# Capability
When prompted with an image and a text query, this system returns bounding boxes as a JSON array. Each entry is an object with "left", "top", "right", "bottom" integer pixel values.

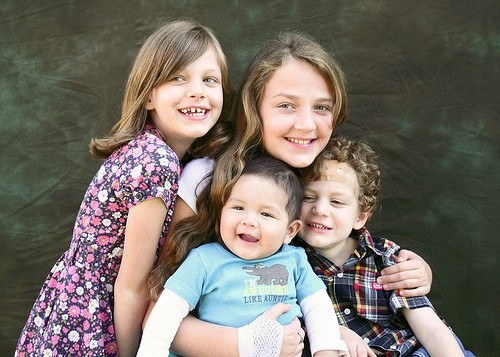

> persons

[
  {"left": 142, "top": 31, "right": 432, "bottom": 357},
  {"left": 136, "top": 159, "right": 349, "bottom": 357},
  {"left": 289, "top": 136, "right": 474, "bottom": 357},
  {"left": 13, "top": 19, "right": 229, "bottom": 357}
]
